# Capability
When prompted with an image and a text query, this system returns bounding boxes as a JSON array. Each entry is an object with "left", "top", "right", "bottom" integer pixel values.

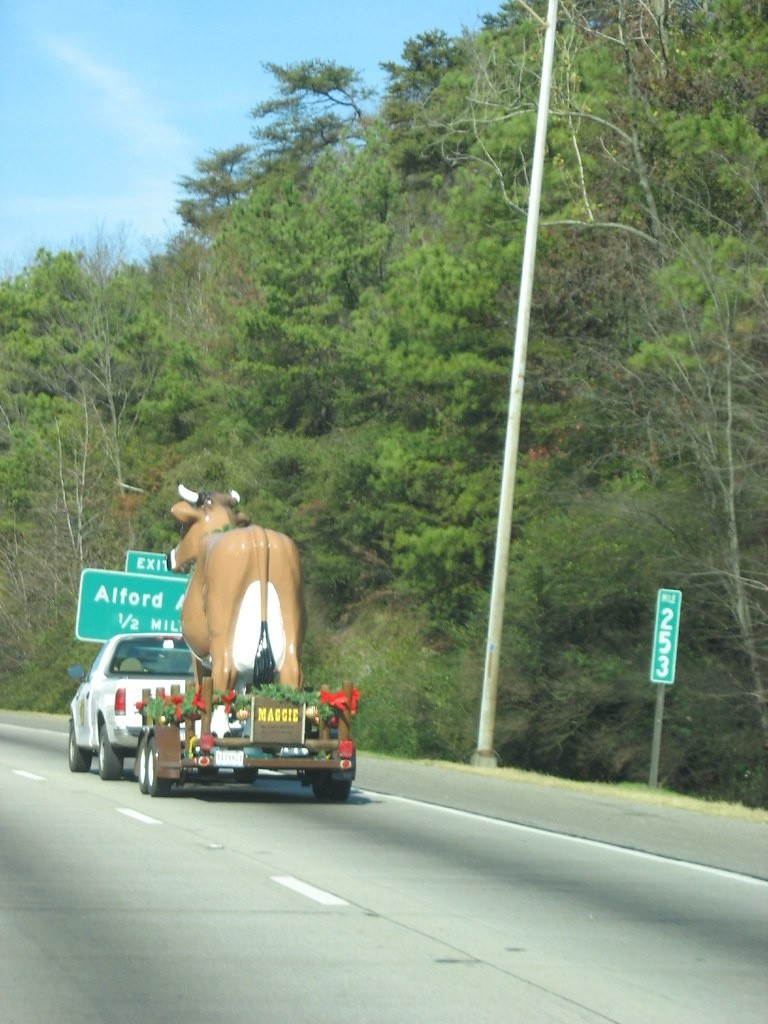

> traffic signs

[{"left": 75, "top": 550, "right": 191, "bottom": 644}]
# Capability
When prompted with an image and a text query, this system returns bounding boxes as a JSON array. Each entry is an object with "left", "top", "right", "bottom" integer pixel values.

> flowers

[{"left": 134, "top": 683, "right": 361, "bottom": 750}]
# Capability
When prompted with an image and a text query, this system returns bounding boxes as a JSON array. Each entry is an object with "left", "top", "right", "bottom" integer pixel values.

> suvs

[{"left": 66, "top": 632, "right": 261, "bottom": 784}]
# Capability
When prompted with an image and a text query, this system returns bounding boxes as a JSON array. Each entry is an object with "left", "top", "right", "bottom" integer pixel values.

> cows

[{"left": 165, "top": 482, "right": 303, "bottom": 692}]
